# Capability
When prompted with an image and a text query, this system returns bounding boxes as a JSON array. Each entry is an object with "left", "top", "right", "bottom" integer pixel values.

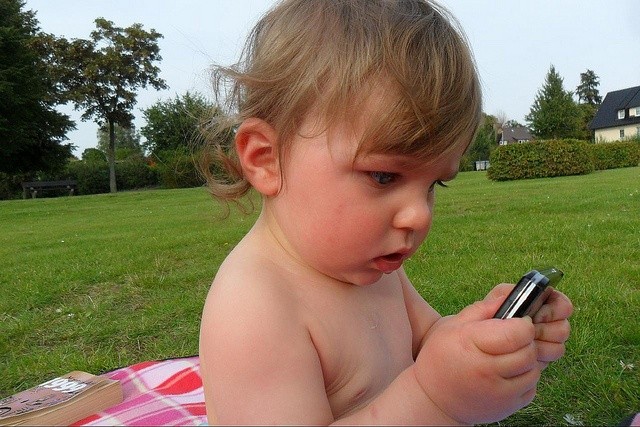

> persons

[{"left": 181, "top": 1, "right": 574, "bottom": 426}]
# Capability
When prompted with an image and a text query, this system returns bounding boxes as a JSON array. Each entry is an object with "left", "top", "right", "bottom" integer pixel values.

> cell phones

[{"left": 492, "top": 267, "right": 564, "bottom": 320}]
